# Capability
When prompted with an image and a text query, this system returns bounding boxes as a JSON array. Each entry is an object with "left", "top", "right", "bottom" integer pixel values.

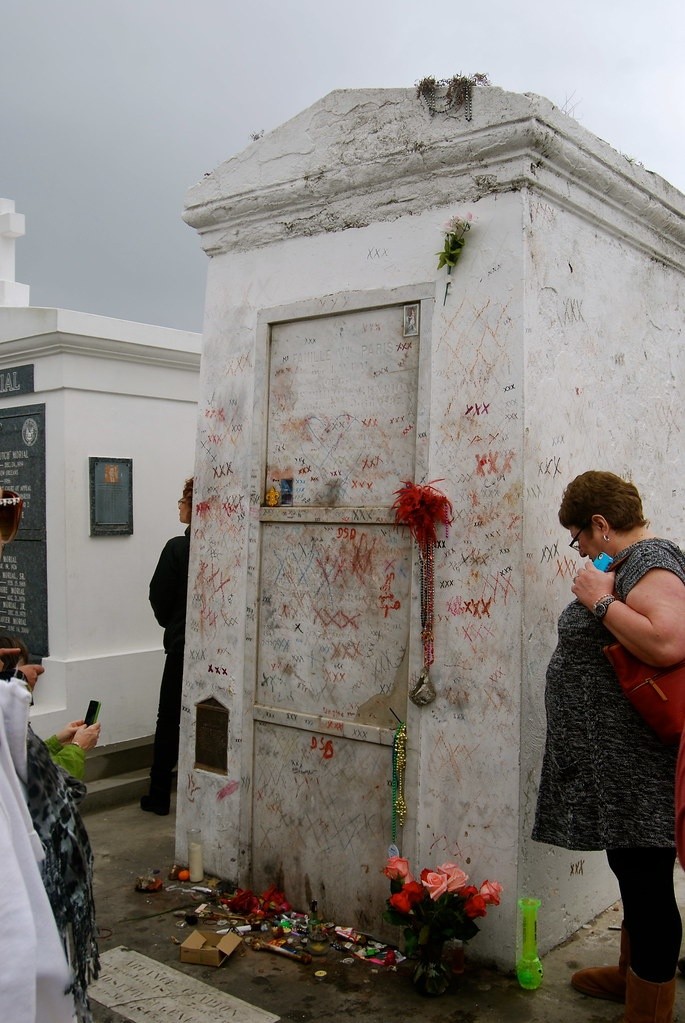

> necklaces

[
  {"left": 388, "top": 722, "right": 408, "bottom": 857},
  {"left": 593, "top": 594, "right": 614, "bottom": 617},
  {"left": 409, "top": 540, "right": 437, "bottom": 706}
]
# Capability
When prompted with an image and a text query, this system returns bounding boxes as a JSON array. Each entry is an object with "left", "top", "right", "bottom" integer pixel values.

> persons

[
  {"left": 0, "top": 488, "right": 102, "bottom": 1023},
  {"left": 140, "top": 478, "right": 195, "bottom": 814},
  {"left": 531, "top": 469, "right": 685, "bottom": 1023}
]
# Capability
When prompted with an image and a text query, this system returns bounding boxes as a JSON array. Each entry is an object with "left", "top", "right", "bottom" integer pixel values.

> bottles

[{"left": 518, "top": 897, "right": 544, "bottom": 990}]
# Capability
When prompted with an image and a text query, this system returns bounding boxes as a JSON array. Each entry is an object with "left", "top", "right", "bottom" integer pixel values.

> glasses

[{"left": 569, "top": 520, "right": 592, "bottom": 551}]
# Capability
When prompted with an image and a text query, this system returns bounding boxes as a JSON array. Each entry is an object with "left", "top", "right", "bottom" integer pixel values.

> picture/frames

[{"left": 89, "top": 456, "right": 134, "bottom": 535}]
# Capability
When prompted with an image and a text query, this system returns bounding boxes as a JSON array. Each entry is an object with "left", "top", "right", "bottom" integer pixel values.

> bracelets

[{"left": 70, "top": 742, "right": 81, "bottom": 748}]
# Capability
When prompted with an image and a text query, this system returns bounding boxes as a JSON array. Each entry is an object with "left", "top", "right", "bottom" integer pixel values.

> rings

[{"left": 572, "top": 575, "right": 579, "bottom": 584}]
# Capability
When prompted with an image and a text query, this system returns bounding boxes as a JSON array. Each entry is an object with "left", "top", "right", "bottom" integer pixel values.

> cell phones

[
  {"left": 28, "top": 655, "right": 42, "bottom": 666},
  {"left": 83, "top": 700, "right": 101, "bottom": 728},
  {"left": 593, "top": 552, "right": 613, "bottom": 572}
]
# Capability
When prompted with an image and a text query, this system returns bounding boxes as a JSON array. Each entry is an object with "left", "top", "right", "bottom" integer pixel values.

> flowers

[
  {"left": 438, "top": 208, "right": 472, "bottom": 305},
  {"left": 383, "top": 855, "right": 502, "bottom": 959}
]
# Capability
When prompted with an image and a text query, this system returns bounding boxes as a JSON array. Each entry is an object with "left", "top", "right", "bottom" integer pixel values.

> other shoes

[
  {"left": 140, "top": 796, "right": 170, "bottom": 815},
  {"left": 625, "top": 966, "right": 675, "bottom": 1023},
  {"left": 572, "top": 919, "right": 629, "bottom": 1001}
]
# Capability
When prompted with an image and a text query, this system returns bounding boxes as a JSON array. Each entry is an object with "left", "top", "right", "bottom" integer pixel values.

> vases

[
  {"left": 514, "top": 897, "right": 543, "bottom": 990},
  {"left": 408, "top": 935, "right": 454, "bottom": 997}
]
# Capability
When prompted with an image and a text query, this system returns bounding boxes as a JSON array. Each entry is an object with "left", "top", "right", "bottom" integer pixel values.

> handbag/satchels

[{"left": 603, "top": 643, "right": 685, "bottom": 736}]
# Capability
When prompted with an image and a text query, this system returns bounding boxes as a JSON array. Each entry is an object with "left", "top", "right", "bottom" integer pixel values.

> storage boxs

[{"left": 180, "top": 929, "right": 243, "bottom": 968}]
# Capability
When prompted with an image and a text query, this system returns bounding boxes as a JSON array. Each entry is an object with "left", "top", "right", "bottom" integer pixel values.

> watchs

[{"left": 594, "top": 597, "right": 619, "bottom": 622}]
